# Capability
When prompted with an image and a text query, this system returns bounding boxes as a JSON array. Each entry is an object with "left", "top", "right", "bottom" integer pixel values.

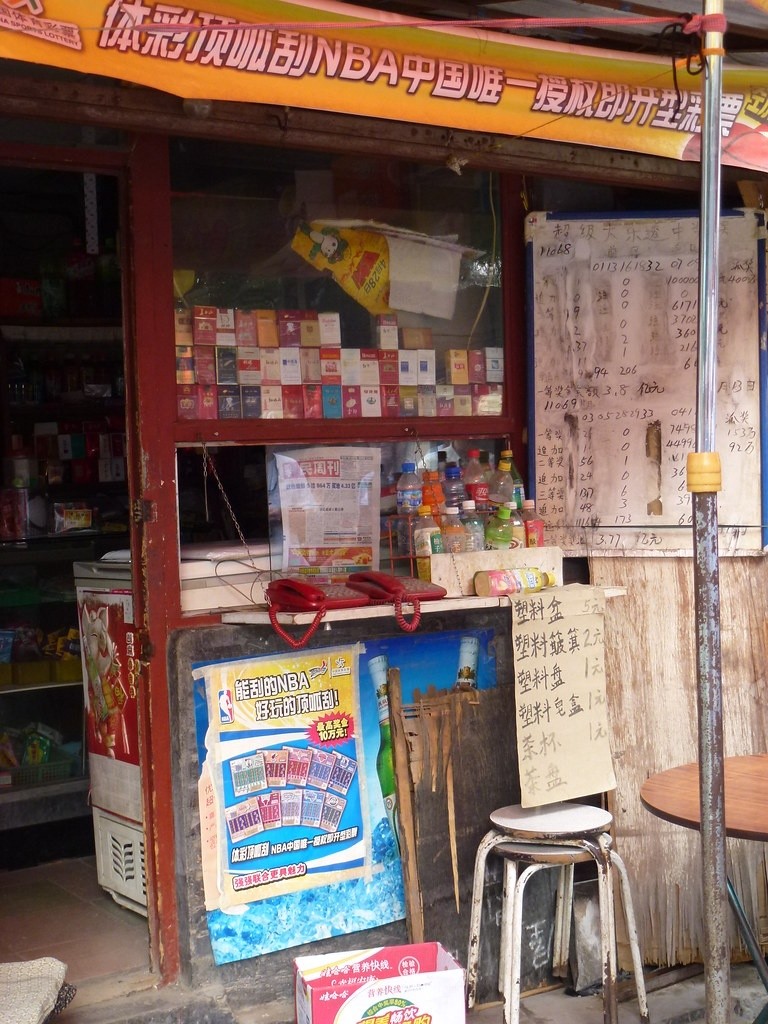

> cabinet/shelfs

[{"left": 0, "top": 317, "right": 130, "bottom": 835}]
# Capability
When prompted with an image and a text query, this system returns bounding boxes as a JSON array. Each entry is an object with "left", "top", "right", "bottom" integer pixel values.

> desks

[{"left": 640, "top": 752, "right": 768, "bottom": 1024}]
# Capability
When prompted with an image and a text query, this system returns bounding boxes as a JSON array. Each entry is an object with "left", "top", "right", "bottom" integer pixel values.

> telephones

[
  {"left": 346, "top": 571, "right": 447, "bottom": 603},
  {"left": 266, "top": 579, "right": 369, "bottom": 612}
]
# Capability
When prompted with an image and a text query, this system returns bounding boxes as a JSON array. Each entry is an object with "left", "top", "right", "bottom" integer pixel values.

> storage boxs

[
  {"left": 415, "top": 545, "right": 564, "bottom": 598},
  {"left": 173, "top": 304, "right": 507, "bottom": 419},
  {"left": 291, "top": 941, "right": 468, "bottom": 1024},
  {"left": 0, "top": 657, "right": 81, "bottom": 691},
  {"left": 0, "top": 746, "right": 75, "bottom": 795}
]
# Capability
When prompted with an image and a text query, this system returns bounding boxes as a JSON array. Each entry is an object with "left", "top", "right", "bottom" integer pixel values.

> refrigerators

[{"left": 73, "top": 537, "right": 414, "bottom": 917}]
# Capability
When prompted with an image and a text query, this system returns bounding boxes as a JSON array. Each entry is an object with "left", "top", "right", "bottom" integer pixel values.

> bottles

[
  {"left": 521, "top": 499, "right": 544, "bottom": 548},
  {"left": 422, "top": 471, "right": 443, "bottom": 522},
  {"left": 502, "top": 450, "right": 526, "bottom": 509},
  {"left": 367, "top": 656, "right": 419, "bottom": 853},
  {"left": 484, "top": 506, "right": 511, "bottom": 549},
  {"left": 444, "top": 467, "right": 466, "bottom": 511},
  {"left": 395, "top": 464, "right": 420, "bottom": 552},
  {"left": 464, "top": 448, "right": 489, "bottom": 510},
  {"left": 460, "top": 499, "right": 484, "bottom": 551},
  {"left": 490, "top": 459, "right": 514, "bottom": 508},
  {"left": 441, "top": 508, "right": 461, "bottom": 553},
  {"left": 476, "top": 567, "right": 555, "bottom": 595},
  {"left": 504, "top": 502, "right": 526, "bottom": 548},
  {"left": 413, "top": 506, "right": 441, "bottom": 582}
]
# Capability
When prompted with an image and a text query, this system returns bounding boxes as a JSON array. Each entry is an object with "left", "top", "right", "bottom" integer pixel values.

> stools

[{"left": 466, "top": 803, "right": 653, "bottom": 1024}]
